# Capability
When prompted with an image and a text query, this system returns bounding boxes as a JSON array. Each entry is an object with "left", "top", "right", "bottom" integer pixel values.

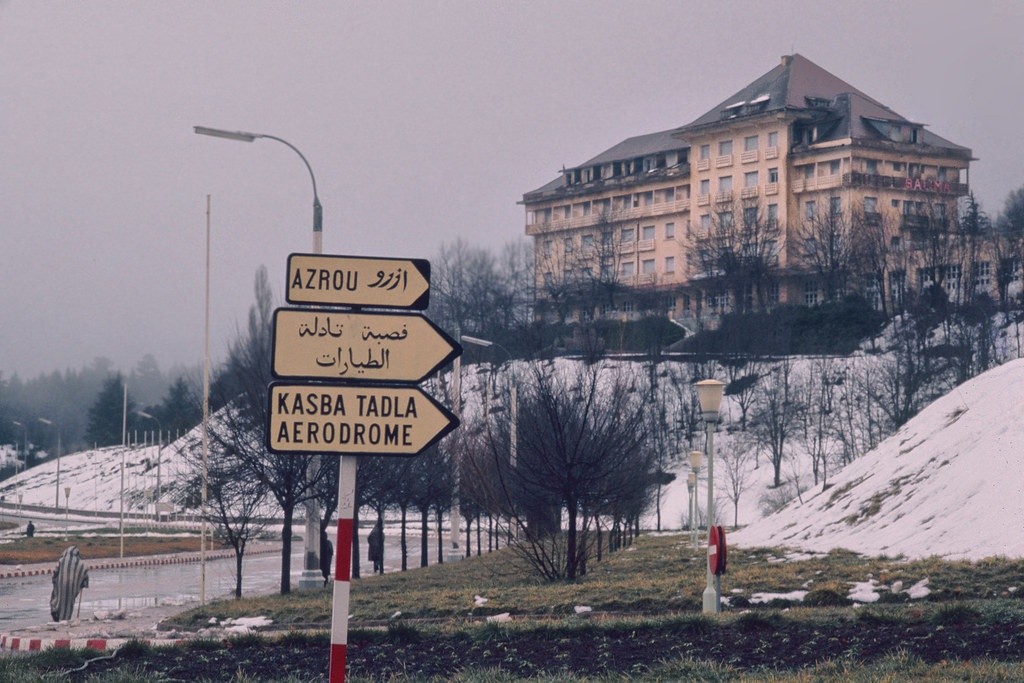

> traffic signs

[{"left": 266, "top": 246, "right": 463, "bottom": 456}]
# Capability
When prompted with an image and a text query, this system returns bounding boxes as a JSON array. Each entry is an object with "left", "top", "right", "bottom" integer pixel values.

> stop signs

[{"left": 706, "top": 524, "right": 721, "bottom": 576}]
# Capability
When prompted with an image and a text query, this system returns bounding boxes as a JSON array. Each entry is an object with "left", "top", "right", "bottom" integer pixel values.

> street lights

[
  {"left": 693, "top": 377, "right": 729, "bottom": 619},
  {"left": 459, "top": 333, "right": 523, "bottom": 552},
  {"left": 6, "top": 406, "right": 169, "bottom": 547},
  {"left": 683, "top": 448, "right": 703, "bottom": 556},
  {"left": 193, "top": 122, "right": 328, "bottom": 592}
]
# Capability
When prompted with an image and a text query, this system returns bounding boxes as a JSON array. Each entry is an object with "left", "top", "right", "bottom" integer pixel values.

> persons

[
  {"left": 320, "top": 531, "right": 334, "bottom": 586},
  {"left": 50, "top": 545, "right": 89, "bottom": 622},
  {"left": 26, "top": 520, "right": 34, "bottom": 537},
  {"left": 368, "top": 523, "right": 385, "bottom": 573}
]
